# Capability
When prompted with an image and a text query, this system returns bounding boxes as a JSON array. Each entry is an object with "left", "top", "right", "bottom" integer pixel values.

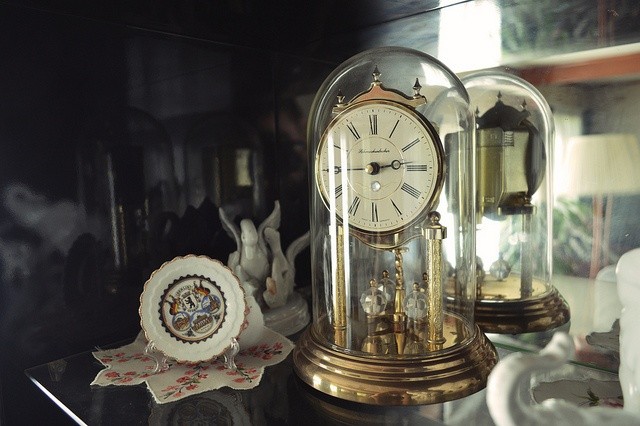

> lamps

[{"left": 557, "top": 134, "right": 639, "bottom": 288}]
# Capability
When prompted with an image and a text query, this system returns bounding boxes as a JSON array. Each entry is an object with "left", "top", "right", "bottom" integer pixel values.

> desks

[{"left": 26, "top": 290, "right": 616, "bottom": 426}]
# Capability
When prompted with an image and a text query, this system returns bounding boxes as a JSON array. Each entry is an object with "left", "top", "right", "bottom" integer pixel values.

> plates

[{"left": 137, "top": 252, "right": 250, "bottom": 367}]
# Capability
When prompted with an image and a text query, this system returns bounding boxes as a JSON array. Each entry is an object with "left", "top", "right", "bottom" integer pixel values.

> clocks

[
  {"left": 422, "top": 70, "right": 570, "bottom": 335},
  {"left": 291, "top": 46, "right": 499, "bottom": 405}
]
